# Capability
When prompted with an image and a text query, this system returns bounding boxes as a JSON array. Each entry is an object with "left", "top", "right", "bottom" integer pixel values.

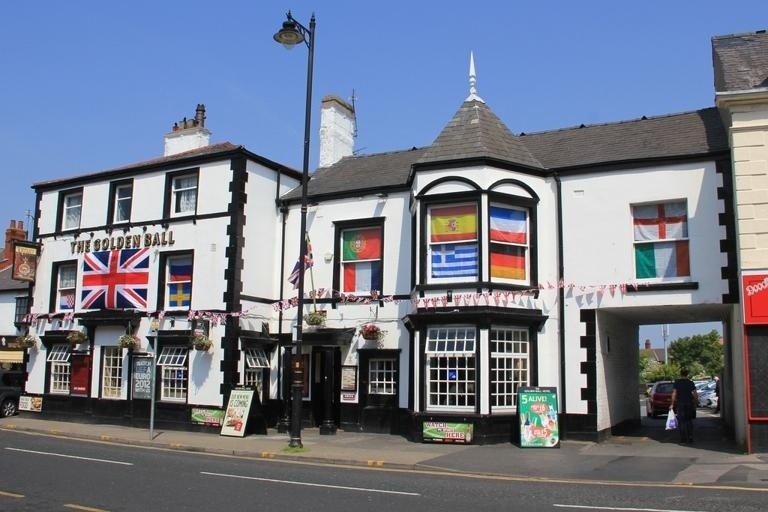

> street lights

[{"left": 273, "top": 9, "right": 316, "bottom": 450}]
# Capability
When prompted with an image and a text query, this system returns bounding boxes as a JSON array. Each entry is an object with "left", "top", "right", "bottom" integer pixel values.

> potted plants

[
  {"left": 192, "top": 334, "right": 213, "bottom": 351},
  {"left": 304, "top": 313, "right": 323, "bottom": 325}
]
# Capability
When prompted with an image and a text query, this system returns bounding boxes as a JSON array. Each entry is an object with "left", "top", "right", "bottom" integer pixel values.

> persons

[
  {"left": 669, "top": 370, "right": 698, "bottom": 445},
  {"left": 712, "top": 374, "right": 723, "bottom": 415}
]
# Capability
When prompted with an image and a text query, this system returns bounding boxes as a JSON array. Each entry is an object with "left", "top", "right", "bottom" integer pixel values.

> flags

[
  {"left": 428, "top": 205, "right": 526, "bottom": 280},
  {"left": 272, "top": 296, "right": 298, "bottom": 313},
  {"left": 342, "top": 226, "right": 383, "bottom": 260},
  {"left": 287, "top": 234, "right": 314, "bottom": 288},
  {"left": 58, "top": 248, "right": 192, "bottom": 312},
  {"left": 343, "top": 263, "right": 383, "bottom": 295},
  {"left": 634, "top": 202, "right": 687, "bottom": 238},
  {"left": 635, "top": 242, "right": 691, "bottom": 278}
]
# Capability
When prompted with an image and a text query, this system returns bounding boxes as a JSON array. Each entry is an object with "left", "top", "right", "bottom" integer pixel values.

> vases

[
  {"left": 18, "top": 341, "right": 33, "bottom": 348},
  {"left": 363, "top": 331, "right": 378, "bottom": 340},
  {"left": 122, "top": 343, "right": 136, "bottom": 348}
]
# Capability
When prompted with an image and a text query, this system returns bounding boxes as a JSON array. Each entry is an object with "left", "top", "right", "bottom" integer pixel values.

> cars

[
  {"left": 646, "top": 381, "right": 698, "bottom": 418},
  {"left": 694, "top": 375, "right": 719, "bottom": 409},
  {"left": 0, "top": 369, "right": 24, "bottom": 416}
]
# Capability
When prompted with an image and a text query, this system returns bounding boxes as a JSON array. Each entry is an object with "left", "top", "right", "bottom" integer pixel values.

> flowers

[
  {"left": 119, "top": 335, "right": 139, "bottom": 346},
  {"left": 359, "top": 323, "right": 381, "bottom": 336},
  {"left": 16, "top": 333, "right": 37, "bottom": 345}
]
